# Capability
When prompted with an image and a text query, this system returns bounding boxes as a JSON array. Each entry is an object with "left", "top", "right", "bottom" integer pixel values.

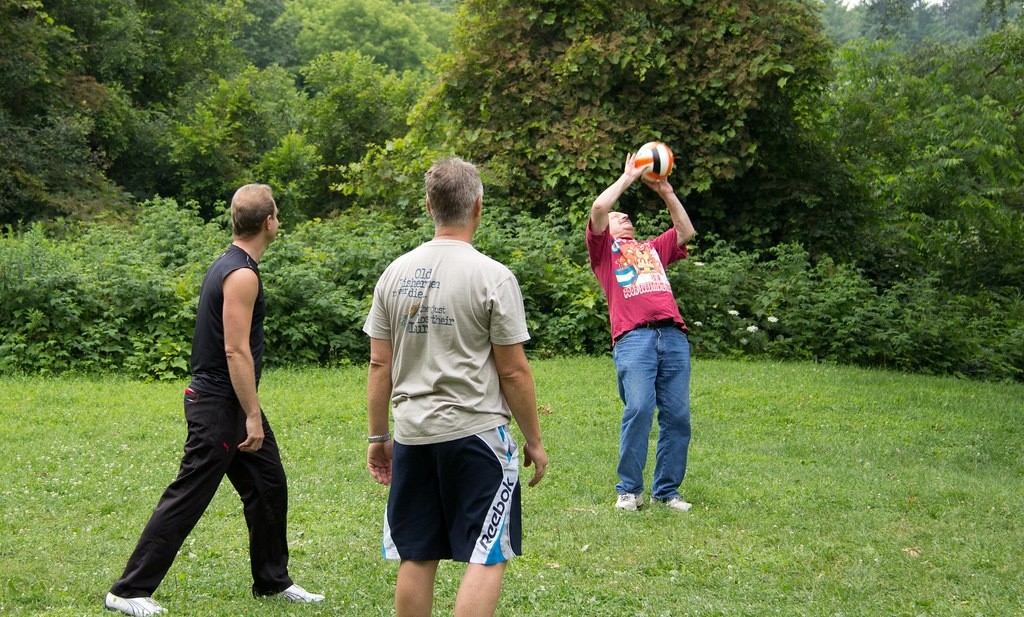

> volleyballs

[{"left": 634, "top": 141, "right": 675, "bottom": 183}]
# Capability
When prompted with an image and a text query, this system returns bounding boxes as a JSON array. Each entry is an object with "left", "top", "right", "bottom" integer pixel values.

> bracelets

[{"left": 369, "top": 434, "right": 391, "bottom": 443}]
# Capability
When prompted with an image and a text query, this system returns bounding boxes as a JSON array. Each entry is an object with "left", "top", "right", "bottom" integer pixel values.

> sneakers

[
  {"left": 253, "top": 583, "right": 326, "bottom": 603},
  {"left": 615, "top": 493, "right": 643, "bottom": 512},
  {"left": 105, "top": 592, "right": 168, "bottom": 617},
  {"left": 649, "top": 496, "right": 692, "bottom": 512}
]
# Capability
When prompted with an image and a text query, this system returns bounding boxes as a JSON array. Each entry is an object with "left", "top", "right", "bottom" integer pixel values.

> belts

[{"left": 637, "top": 321, "right": 674, "bottom": 329}]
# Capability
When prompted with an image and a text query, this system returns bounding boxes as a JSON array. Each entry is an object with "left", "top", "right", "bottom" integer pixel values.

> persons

[
  {"left": 103, "top": 184, "right": 325, "bottom": 617},
  {"left": 364, "top": 157, "right": 547, "bottom": 617},
  {"left": 586, "top": 152, "right": 696, "bottom": 512}
]
